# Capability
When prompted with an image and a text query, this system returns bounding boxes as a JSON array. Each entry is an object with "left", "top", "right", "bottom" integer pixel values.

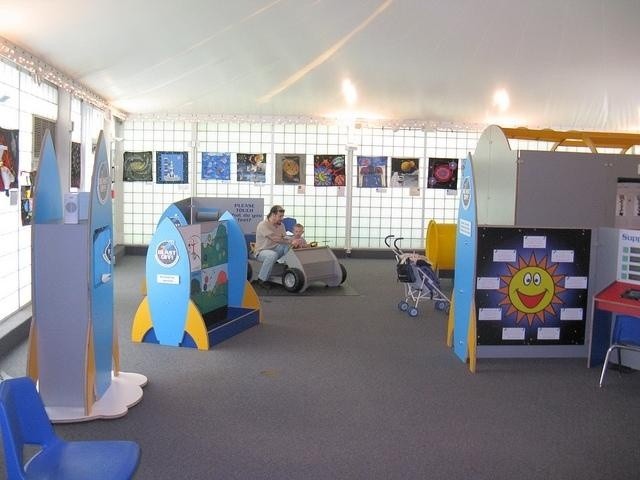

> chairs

[
  {"left": 0, "top": 375, "right": 140, "bottom": 480},
  {"left": 598, "top": 314, "right": 640, "bottom": 387}
]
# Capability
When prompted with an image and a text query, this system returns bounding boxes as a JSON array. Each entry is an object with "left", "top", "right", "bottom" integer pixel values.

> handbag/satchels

[{"left": 397, "top": 263, "right": 416, "bottom": 283}]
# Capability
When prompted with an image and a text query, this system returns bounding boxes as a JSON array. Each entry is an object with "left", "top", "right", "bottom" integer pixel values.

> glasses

[{"left": 275, "top": 209, "right": 285, "bottom": 212}]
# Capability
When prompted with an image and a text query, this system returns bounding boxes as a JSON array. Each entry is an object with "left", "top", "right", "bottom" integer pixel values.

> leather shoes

[{"left": 257, "top": 279, "right": 272, "bottom": 290}]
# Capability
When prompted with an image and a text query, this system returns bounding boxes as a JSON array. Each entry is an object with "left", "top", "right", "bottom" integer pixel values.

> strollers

[{"left": 385, "top": 235, "right": 455, "bottom": 321}]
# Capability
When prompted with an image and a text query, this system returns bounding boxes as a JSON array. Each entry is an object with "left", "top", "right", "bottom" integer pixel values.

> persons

[{"left": 254, "top": 205, "right": 308, "bottom": 290}]
